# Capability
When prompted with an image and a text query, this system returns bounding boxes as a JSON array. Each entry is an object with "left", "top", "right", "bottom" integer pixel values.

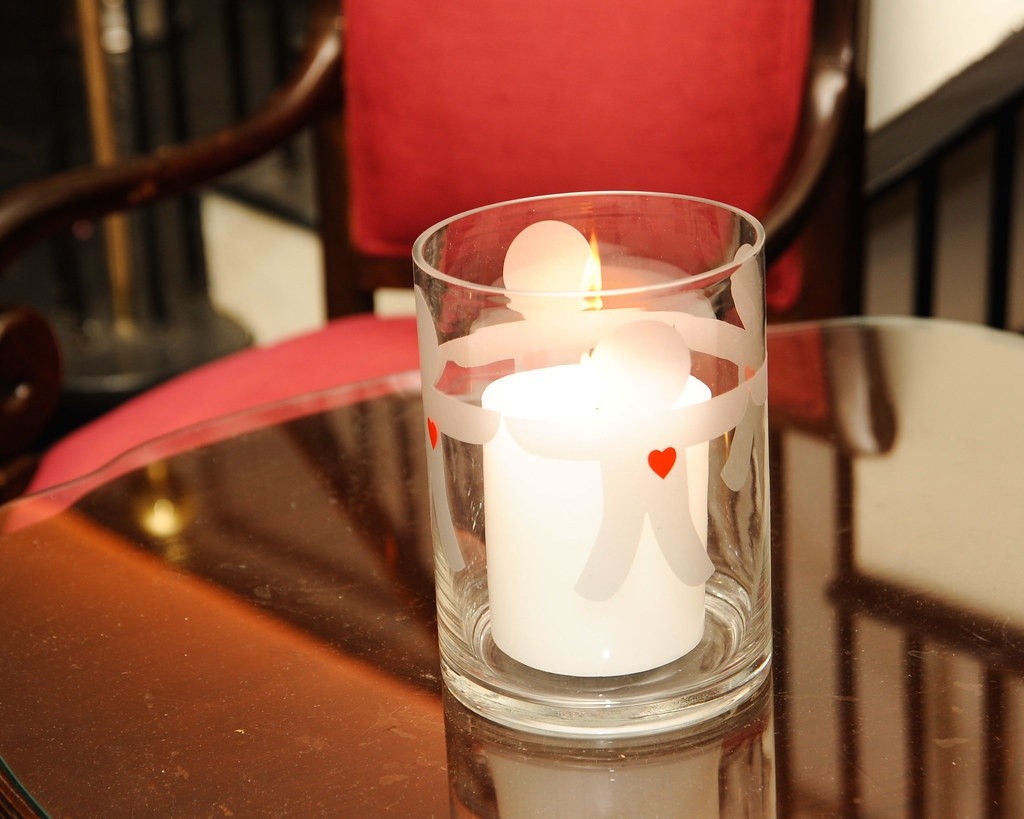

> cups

[{"left": 413, "top": 190, "right": 771, "bottom": 738}]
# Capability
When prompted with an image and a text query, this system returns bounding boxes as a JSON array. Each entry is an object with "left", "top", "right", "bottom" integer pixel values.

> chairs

[{"left": 0, "top": 0, "right": 870, "bottom": 536}]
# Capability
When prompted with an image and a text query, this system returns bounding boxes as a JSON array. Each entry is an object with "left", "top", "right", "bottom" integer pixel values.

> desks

[{"left": 0, "top": 312, "right": 1024, "bottom": 817}]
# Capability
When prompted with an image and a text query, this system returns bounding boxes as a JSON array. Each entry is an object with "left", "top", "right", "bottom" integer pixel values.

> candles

[{"left": 484, "top": 229, "right": 718, "bottom": 680}]
